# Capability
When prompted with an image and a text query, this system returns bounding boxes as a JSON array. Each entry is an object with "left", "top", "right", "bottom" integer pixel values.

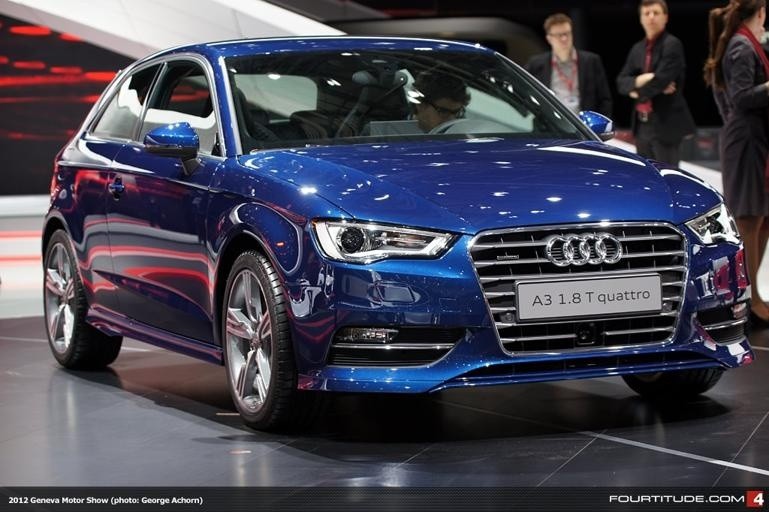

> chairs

[
  {"left": 337, "top": 85, "right": 409, "bottom": 136},
  {"left": 202, "top": 87, "right": 280, "bottom": 142},
  {"left": 288, "top": 110, "right": 337, "bottom": 139}
]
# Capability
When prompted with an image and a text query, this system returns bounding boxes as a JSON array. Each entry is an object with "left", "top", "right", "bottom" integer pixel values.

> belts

[{"left": 637, "top": 110, "right": 649, "bottom": 123}]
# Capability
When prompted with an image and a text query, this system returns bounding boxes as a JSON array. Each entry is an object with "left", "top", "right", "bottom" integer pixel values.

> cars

[{"left": 42, "top": 36, "right": 755, "bottom": 431}]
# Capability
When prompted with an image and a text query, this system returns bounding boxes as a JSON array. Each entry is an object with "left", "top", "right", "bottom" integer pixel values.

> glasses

[{"left": 431, "top": 102, "right": 466, "bottom": 119}]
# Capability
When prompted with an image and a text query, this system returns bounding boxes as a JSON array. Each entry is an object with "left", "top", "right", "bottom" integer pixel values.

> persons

[
  {"left": 523, "top": 10, "right": 613, "bottom": 130},
  {"left": 702, "top": 0, "right": 768, "bottom": 329},
  {"left": 403, "top": 63, "right": 474, "bottom": 135},
  {"left": 615, "top": 1, "right": 698, "bottom": 168}
]
